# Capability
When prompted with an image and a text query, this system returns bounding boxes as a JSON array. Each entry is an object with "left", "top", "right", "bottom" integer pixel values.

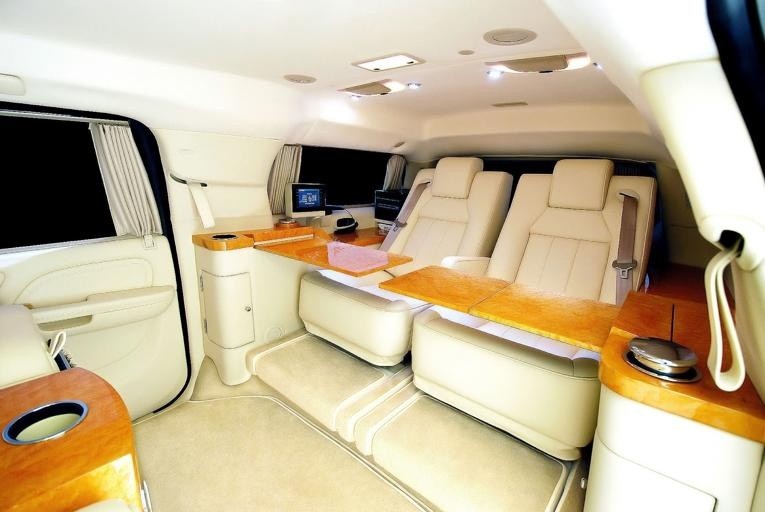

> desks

[
  {"left": 253, "top": 225, "right": 413, "bottom": 276},
  {"left": 378, "top": 265, "right": 622, "bottom": 352}
]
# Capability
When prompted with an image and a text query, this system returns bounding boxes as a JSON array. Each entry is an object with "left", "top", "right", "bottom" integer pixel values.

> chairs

[
  {"left": 410, "top": 159, "right": 657, "bottom": 462},
  {"left": 299, "top": 156, "right": 514, "bottom": 366},
  {"left": 0, "top": 304, "right": 71, "bottom": 398}
]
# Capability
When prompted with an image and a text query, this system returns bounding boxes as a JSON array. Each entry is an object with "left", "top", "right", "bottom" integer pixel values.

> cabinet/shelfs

[
  {"left": 577, "top": 428, "right": 718, "bottom": 512},
  {"left": 201, "top": 271, "right": 256, "bottom": 349}
]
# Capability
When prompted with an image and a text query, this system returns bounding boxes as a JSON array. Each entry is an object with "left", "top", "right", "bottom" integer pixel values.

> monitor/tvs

[{"left": 285, "top": 182, "right": 326, "bottom": 218}]
